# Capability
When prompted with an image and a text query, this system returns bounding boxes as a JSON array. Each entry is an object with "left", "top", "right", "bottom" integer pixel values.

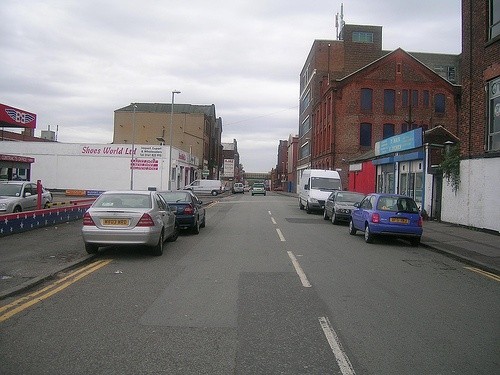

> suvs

[{"left": 251, "top": 183, "right": 266, "bottom": 196}]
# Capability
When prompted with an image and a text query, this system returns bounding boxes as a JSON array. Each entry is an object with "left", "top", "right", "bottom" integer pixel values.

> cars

[
  {"left": 157, "top": 189, "right": 206, "bottom": 235},
  {"left": 323, "top": 191, "right": 365, "bottom": 225},
  {"left": 81, "top": 190, "right": 179, "bottom": 256},
  {"left": 0, "top": 180, "right": 53, "bottom": 214},
  {"left": 349, "top": 193, "right": 423, "bottom": 247},
  {"left": 243, "top": 185, "right": 251, "bottom": 192}
]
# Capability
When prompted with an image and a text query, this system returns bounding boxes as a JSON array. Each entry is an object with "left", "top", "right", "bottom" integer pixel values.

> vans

[
  {"left": 298, "top": 169, "right": 347, "bottom": 214},
  {"left": 234, "top": 183, "right": 245, "bottom": 194},
  {"left": 184, "top": 180, "right": 223, "bottom": 196}
]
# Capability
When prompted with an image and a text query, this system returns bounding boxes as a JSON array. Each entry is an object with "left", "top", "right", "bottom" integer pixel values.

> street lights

[{"left": 168, "top": 90, "right": 182, "bottom": 190}]
